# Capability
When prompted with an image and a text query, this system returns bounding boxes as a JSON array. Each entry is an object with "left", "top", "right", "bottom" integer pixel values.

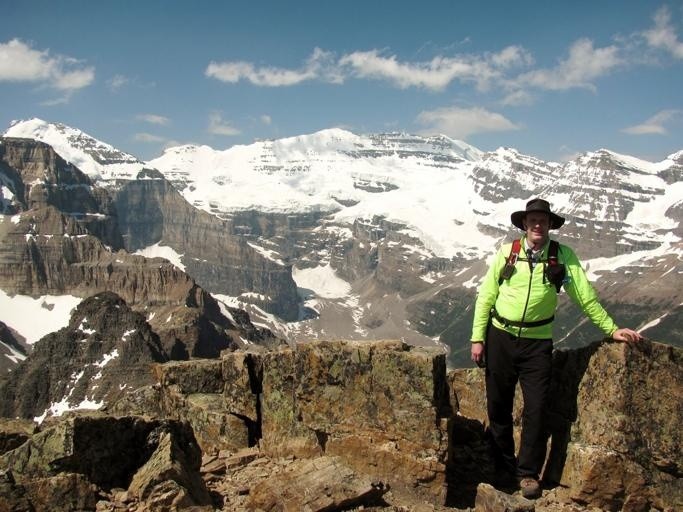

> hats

[{"left": 509, "top": 198, "right": 565, "bottom": 231}]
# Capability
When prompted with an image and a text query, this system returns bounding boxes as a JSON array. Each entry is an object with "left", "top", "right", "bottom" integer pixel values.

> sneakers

[{"left": 519, "top": 475, "right": 541, "bottom": 498}]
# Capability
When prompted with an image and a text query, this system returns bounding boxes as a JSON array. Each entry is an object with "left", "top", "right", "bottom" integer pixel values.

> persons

[{"left": 469, "top": 197, "right": 645, "bottom": 500}]
